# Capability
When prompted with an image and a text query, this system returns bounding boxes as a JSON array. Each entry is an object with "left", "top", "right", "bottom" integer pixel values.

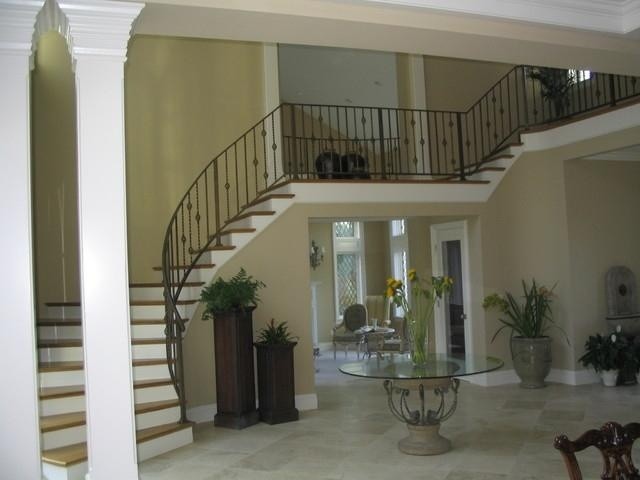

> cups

[{"left": 371, "top": 318, "right": 378, "bottom": 330}]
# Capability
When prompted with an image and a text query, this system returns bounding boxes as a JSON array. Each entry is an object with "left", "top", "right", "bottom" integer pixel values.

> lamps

[{"left": 309, "top": 240, "right": 325, "bottom": 270}]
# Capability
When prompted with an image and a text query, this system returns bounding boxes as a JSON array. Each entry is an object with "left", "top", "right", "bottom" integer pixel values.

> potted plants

[
  {"left": 253, "top": 317, "right": 299, "bottom": 425},
  {"left": 200, "top": 266, "right": 267, "bottom": 430}
]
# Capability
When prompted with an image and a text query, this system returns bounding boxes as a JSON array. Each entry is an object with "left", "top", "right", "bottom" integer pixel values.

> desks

[{"left": 337, "top": 352, "right": 505, "bottom": 457}]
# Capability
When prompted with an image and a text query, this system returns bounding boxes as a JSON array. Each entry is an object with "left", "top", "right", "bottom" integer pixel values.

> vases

[
  {"left": 407, "top": 318, "right": 428, "bottom": 368},
  {"left": 511, "top": 335, "right": 553, "bottom": 388},
  {"left": 599, "top": 368, "right": 620, "bottom": 386}
]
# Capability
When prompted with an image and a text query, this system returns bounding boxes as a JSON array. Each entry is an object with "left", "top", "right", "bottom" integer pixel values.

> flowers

[
  {"left": 384, "top": 268, "right": 454, "bottom": 365},
  {"left": 577, "top": 324, "right": 640, "bottom": 374},
  {"left": 483, "top": 277, "right": 570, "bottom": 359}
]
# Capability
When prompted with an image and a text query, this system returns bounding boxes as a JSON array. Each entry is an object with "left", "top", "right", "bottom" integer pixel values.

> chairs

[
  {"left": 553, "top": 421, "right": 640, "bottom": 480},
  {"left": 330, "top": 303, "right": 369, "bottom": 361},
  {"left": 379, "top": 317, "right": 408, "bottom": 353}
]
